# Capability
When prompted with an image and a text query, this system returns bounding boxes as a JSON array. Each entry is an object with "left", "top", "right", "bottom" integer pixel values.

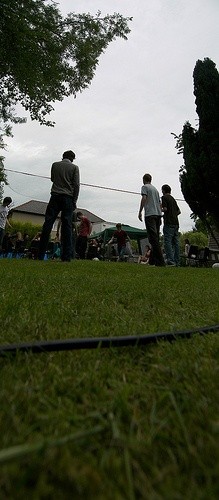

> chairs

[
  {"left": 181, "top": 246, "right": 210, "bottom": 267},
  {"left": 2, "top": 234, "right": 61, "bottom": 259}
]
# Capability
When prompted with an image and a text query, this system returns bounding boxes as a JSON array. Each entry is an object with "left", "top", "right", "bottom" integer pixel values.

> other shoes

[{"left": 166, "top": 260, "right": 179, "bottom": 267}]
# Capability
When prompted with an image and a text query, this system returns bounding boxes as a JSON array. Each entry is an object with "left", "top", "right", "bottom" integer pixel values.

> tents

[{"left": 89, "top": 224, "right": 148, "bottom": 261}]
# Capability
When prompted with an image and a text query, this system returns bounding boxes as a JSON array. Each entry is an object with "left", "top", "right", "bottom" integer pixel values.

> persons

[
  {"left": 2, "top": 231, "right": 104, "bottom": 262},
  {"left": 55, "top": 213, "right": 77, "bottom": 259},
  {"left": 184, "top": 239, "right": 191, "bottom": 266},
  {"left": 75, "top": 212, "right": 92, "bottom": 259},
  {"left": 36, "top": 151, "right": 79, "bottom": 261},
  {"left": 0, "top": 197, "right": 13, "bottom": 257},
  {"left": 138, "top": 245, "right": 151, "bottom": 264},
  {"left": 160, "top": 185, "right": 181, "bottom": 267},
  {"left": 139, "top": 174, "right": 166, "bottom": 267},
  {"left": 108, "top": 247, "right": 116, "bottom": 262},
  {"left": 104, "top": 223, "right": 131, "bottom": 262}
]
